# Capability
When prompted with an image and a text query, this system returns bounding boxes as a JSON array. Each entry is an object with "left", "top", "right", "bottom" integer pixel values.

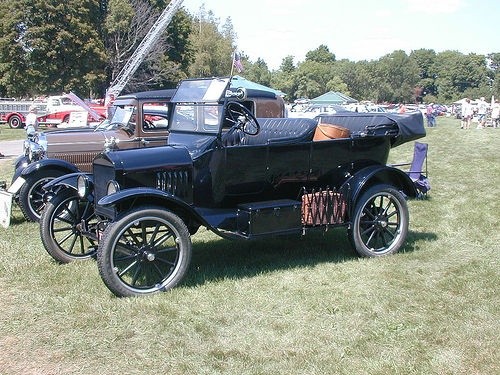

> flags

[{"left": 233, "top": 52, "right": 245, "bottom": 73}]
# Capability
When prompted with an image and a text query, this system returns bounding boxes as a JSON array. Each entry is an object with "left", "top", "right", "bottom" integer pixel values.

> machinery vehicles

[{"left": 88, "top": 0, "right": 183, "bottom": 122}]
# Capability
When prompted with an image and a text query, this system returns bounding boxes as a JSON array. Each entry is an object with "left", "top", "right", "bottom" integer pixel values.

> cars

[
  {"left": 367, "top": 101, "right": 493, "bottom": 122},
  {"left": 40, "top": 76, "right": 426, "bottom": 299},
  {"left": 16, "top": 90, "right": 288, "bottom": 219}
]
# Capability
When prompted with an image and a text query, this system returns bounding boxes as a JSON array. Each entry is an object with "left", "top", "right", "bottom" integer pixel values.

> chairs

[{"left": 388, "top": 141, "right": 432, "bottom": 198}]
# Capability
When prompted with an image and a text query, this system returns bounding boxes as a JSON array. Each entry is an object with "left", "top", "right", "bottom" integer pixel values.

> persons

[
  {"left": 489, "top": 98, "right": 500, "bottom": 128},
  {"left": 24, "top": 106, "right": 40, "bottom": 143},
  {"left": 361, "top": 96, "right": 475, "bottom": 130},
  {"left": 476, "top": 97, "right": 490, "bottom": 130}
]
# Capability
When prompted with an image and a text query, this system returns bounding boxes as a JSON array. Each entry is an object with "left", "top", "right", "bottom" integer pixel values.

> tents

[
  {"left": 307, "top": 91, "right": 346, "bottom": 104},
  {"left": 336, "top": 92, "right": 359, "bottom": 103},
  {"left": 451, "top": 97, "right": 478, "bottom": 115},
  {"left": 228, "top": 75, "right": 287, "bottom": 97}
]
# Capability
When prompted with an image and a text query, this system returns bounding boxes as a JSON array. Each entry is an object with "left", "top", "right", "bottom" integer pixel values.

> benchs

[
  {"left": 312, "top": 111, "right": 398, "bottom": 142},
  {"left": 219, "top": 118, "right": 318, "bottom": 149}
]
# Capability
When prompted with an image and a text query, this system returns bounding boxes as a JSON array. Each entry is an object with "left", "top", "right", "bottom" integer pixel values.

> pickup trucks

[{"left": 0, "top": 95, "right": 86, "bottom": 130}]
